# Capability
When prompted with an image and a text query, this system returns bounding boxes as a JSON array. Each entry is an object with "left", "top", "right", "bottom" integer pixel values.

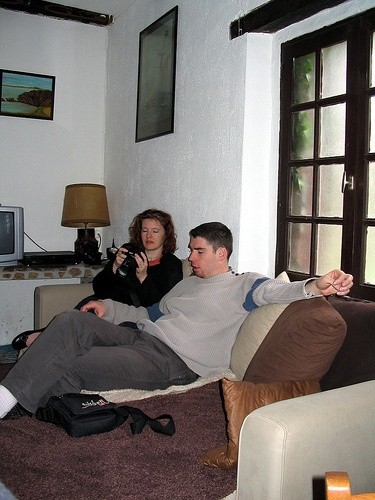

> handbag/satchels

[{"left": 35, "top": 393, "right": 175, "bottom": 438}]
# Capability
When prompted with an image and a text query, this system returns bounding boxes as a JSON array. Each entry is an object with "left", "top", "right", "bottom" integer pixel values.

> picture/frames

[
  {"left": 135, "top": 5, "right": 178, "bottom": 143},
  {"left": 0, "top": 69, "right": 56, "bottom": 120}
]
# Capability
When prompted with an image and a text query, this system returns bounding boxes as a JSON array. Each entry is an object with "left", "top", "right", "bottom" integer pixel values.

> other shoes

[{"left": 12, "top": 328, "right": 47, "bottom": 350}]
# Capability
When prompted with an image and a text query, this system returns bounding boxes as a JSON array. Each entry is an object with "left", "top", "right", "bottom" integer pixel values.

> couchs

[{"left": 0, "top": 282, "right": 375, "bottom": 500}]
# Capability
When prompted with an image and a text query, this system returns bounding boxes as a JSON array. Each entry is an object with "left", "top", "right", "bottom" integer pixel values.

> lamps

[{"left": 61, "top": 184, "right": 110, "bottom": 265}]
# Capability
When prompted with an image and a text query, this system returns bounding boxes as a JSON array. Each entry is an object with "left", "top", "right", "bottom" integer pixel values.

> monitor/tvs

[{"left": 0, "top": 205, "right": 24, "bottom": 267}]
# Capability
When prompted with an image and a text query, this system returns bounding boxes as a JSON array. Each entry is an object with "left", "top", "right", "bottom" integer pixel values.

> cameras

[{"left": 117, "top": 243, "right": 147, "bottom": 278}]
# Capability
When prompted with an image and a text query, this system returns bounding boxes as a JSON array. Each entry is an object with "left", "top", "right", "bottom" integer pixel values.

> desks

[{"left": 0, "top": 261, "right": 111, "bottom": 283}]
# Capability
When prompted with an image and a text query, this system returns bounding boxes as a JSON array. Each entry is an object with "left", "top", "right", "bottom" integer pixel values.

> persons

[
  {"left": 0, "top": 222, "right": 353, "bottom": 421},
  {"left": 11, "top": 208, "right": 183, "bottom": 351}
]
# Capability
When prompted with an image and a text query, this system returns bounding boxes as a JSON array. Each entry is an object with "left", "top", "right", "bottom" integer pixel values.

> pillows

[
  {"left": 229, "top": 272, "right": 294, "bottom": 380},
  {"left": 241, "top": 297, "right": 347, "bottom": 383},
  {"left": 201, "top": 377, "right": 321, "bottom": 468}
]
